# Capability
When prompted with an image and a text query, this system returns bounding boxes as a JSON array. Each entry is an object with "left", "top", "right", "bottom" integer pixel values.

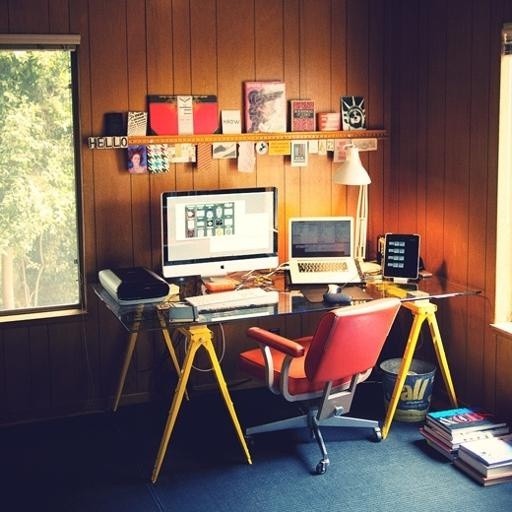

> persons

[{"left": 128, "top": 149, "right": 147, "bottom": 174}]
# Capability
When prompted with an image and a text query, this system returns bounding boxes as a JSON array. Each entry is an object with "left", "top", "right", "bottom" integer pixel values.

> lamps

[{"left": 330, "top": 142, "right": 373, "bottom": 262}]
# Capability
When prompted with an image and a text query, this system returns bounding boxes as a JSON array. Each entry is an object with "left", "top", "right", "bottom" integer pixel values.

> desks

[{"left": 88, "top": 262, "right": 483, "bottom": 486}]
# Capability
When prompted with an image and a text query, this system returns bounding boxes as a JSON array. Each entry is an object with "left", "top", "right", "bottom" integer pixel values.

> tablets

[{"left": 382, "top": 233, "right": 421, "bottom": 279}]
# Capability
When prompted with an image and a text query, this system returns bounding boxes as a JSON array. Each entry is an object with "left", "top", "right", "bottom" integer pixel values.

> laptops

[{"left": 288, "top": 216, "right": 362, "bottom": 285}]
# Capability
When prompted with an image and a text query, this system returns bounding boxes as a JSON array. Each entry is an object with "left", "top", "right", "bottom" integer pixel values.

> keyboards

[{"left": 184, "top": 287, "right": 280, "bottom": 313}]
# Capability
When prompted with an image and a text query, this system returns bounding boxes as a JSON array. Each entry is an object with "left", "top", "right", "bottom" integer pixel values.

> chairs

[{"left": 233, "top": 297, "right": 402, "bottom": 474}]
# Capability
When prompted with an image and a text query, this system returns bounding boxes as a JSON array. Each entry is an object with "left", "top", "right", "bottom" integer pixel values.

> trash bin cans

[{"left": 380, "top": 357, "right": 437, "bottom": 423}]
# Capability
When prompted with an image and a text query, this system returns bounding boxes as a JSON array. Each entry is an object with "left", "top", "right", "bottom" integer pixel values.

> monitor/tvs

[{"left": 160, "top": 186, "right": 279, "bottom": 288}]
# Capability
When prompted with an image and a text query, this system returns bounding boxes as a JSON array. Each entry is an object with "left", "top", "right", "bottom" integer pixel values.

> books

[
  {"left": 221, "top": 109, "right": 241, "bottom": 136},
  {"left": 244, "top": 78, "right": 288, "bottom": 133},
  {"left": 318, "top": 112, "right": 341, "bottom": 130},
  {"left": 339, "top": 95, "right": 368, "bottom": 132},
  {"left": 103, "top": 111, "right": 127, "bottom": 138},
  {"left": 419, "top": 405, "right": 512, "bottom": 488},
  {"left": 146, "top": 94, "right": 220, "bottom": 136},
  {"left": 290, "top": 98, "right": 317, "bottom": 132},
  {"left": 125, "top": 111, "right": 149, "bottom": 136}
]
